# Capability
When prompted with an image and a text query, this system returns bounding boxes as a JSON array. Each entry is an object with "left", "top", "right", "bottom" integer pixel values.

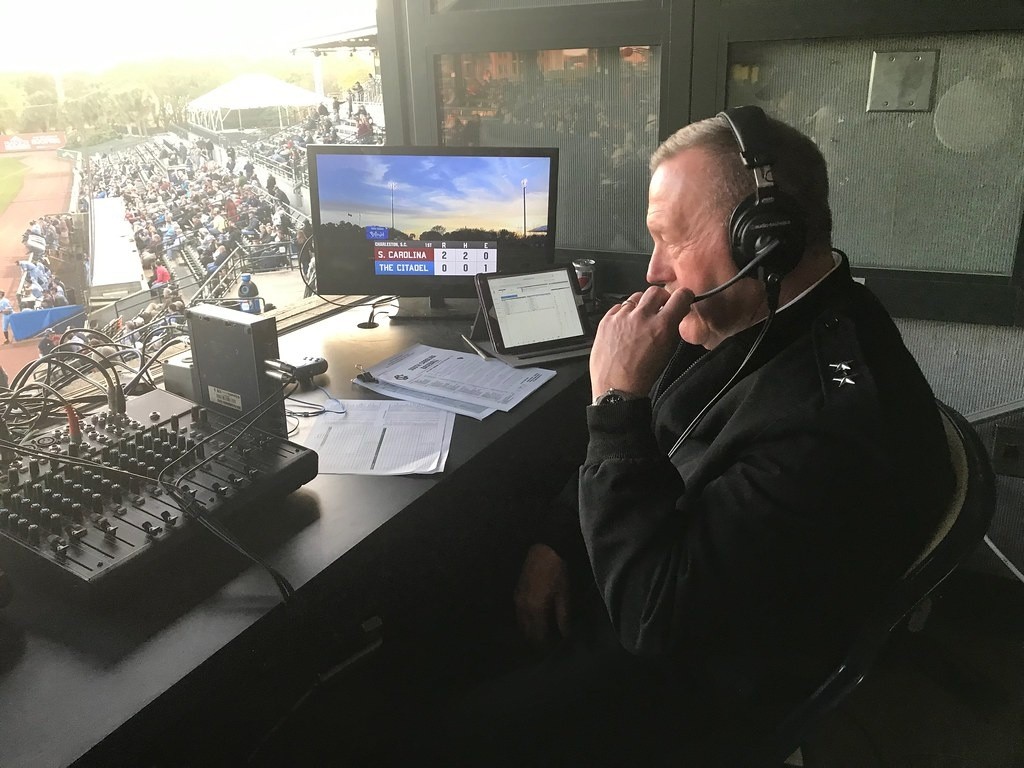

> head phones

[{"left": 719, "top": 104, "right": 809, "bottom": 284}]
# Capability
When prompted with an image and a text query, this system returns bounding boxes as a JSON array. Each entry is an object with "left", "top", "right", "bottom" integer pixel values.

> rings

[{"left": 620, "top": 300, "right": 637, "bottom": 309}]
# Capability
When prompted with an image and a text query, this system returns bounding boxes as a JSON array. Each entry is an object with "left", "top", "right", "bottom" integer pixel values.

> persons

[
  {"left": 459, "top": 115, "right": 955, "bottom": 767},
  {"left": 244, "top": 73, "right": 383, "bottom": 181},
  {"left": 437, "top": 70, "right": 659, "bottom": 201},
  {"left": 0, "top": 136, "right": 315, "bottom": 368}
]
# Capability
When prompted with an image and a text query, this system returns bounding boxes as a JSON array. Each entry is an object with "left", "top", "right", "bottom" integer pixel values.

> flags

[{"left": 348, "top": 213, "right": 352, "bottom": 217}]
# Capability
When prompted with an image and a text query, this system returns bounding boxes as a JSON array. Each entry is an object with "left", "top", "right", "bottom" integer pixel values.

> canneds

[{"left": 572, "top": 259, "right": 595, "bottom": 302}]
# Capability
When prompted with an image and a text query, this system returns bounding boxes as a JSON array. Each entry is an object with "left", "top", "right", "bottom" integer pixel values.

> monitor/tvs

[{"left": 306, "top": 145, "right": 560, "bottom": 320}]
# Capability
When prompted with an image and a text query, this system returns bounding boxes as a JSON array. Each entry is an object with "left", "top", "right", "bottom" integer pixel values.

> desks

[{"left": 0, "top": 285, "right": 626, "bottom": 768}]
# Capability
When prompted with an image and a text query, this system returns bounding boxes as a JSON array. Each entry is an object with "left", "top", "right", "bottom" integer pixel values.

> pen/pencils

[{"left": 459, "top": 331, "right": 488, "bottom": 360}]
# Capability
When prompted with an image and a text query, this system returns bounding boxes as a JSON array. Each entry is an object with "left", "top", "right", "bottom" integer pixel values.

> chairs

[{"left": 743, "top": 395, "right": 996, "bottom": 768}]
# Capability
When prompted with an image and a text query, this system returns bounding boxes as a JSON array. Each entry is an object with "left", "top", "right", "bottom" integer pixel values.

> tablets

[{"left": 473, "top": 264, "right": 596, "bottom": 353}]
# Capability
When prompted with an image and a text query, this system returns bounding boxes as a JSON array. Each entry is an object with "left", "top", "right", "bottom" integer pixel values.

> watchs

[{"left": 592, "top": 388, "right": 635, "bottom": 406}]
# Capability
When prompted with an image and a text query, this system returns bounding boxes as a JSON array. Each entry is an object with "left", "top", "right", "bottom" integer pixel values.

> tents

[{"left": 185, "top": 72, "right": 324, "bottom": 140}]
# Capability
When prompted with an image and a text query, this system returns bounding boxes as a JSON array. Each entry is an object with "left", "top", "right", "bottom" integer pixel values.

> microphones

[{"left": 694, "top": 257, "right": 761, "bottom": 302}]
